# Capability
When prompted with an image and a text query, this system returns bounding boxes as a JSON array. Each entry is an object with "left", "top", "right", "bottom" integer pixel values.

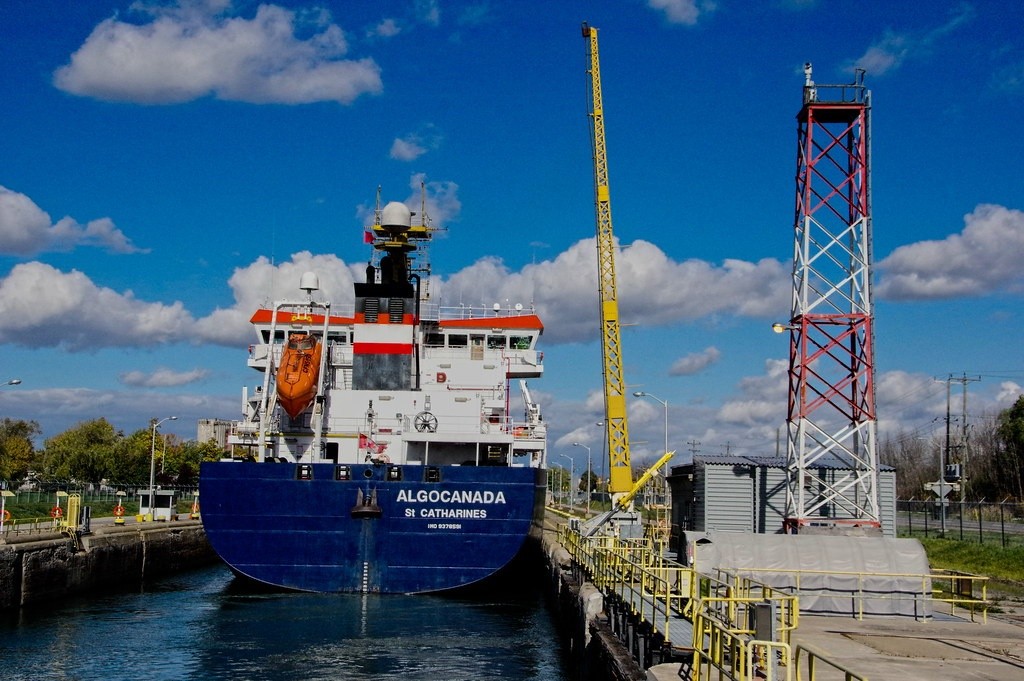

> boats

[{"left": 199, "top": 181, "right": 547, "bottom": 595}]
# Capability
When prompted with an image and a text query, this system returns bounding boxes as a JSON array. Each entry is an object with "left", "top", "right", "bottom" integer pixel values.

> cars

[{"left": 21, "top": 481, "right": 36, "bottom": 490}]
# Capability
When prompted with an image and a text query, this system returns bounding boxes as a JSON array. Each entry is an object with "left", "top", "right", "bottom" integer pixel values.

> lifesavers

[
  {"left": 0, "top": 510, "right": 11, "bottom": 523},
  {"left": 191, "top": 503, "right": 199, "bottom": 512},
  {"left": 378, "top": 454, "right": 390, "bottom": 463},
  {"left": 50, "top": 507, "right": 63, "bottom": 519},
  {"left": 113, "top": 506, "right": 125, "bottom": 517}
]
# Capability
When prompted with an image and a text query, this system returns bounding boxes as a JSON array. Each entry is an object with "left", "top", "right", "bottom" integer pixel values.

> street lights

[
  {"left": 633, "top": 390, "right": 669, "bottom": 505},
  {"left": 547, "top": 467, "right": 556, "bottom": 507},
  {"left": 916, "top": 435, "right": 950, "bottom": 532},
  {"left": 573, "top": 442, "right": 592, "bottom": 519},
  {"left": 147, "top": 416, "right": 178, "bottom": 522},
  {"left": 771, "top": 320, "right": 807, "bottom": 536},
  {"left": 552, "top": 461, "right": 564, "bottom": 510},
  {"left": 596, "top": 421, "right": 606, "bottom": 512},
  {"left": 560, "top": 453, "right": 575, "bottom": 514}
]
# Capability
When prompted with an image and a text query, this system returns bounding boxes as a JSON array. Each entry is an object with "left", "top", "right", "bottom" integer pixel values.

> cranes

[{"left": 577, "top": 16, "right": 647, "bottom": 545}]
